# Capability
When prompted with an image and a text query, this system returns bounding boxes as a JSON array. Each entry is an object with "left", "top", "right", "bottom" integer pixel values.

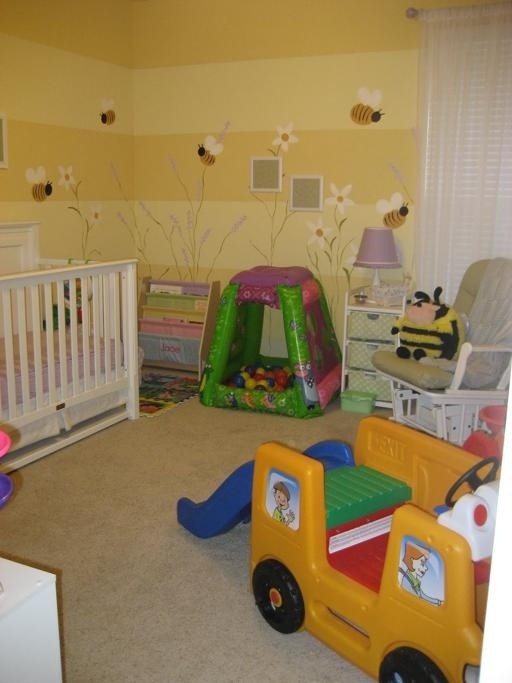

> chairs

[{"left": 371, "top": 258, "right": 510, "bottom": 446}]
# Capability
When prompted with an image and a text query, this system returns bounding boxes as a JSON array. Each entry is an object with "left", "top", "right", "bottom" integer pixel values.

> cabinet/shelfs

[
  {"left": 342, "top": 286, "right": 408, "bottom": 412},
  {"left": 139, "top": 278, "right": 217, "bottom": 381}
]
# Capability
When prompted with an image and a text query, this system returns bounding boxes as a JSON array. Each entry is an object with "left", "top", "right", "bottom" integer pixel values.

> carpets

[{"left": 138, "top": 370, "right": 202, "bottom": 420}]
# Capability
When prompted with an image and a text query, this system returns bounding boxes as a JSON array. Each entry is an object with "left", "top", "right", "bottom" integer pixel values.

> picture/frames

[
  {"left": 250, "top": 158, "right": 282, "bottom": 191},
  {"left": 290, "top": 176, "right": 323, "bottom": 211}
]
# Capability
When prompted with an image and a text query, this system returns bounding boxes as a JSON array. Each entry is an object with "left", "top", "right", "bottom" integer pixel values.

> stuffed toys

[{"left": 388, "top": 284, "right": 466, "bottom": 362}]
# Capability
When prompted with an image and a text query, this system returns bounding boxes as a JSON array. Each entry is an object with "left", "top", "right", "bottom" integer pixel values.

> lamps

[{"left": 354, "top": 227, "right": 403, "bottom": 304}]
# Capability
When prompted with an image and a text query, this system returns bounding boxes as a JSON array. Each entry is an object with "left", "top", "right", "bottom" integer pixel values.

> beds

[{"left": 1, "top": 257, "right": 138, "bottom": 476}]
